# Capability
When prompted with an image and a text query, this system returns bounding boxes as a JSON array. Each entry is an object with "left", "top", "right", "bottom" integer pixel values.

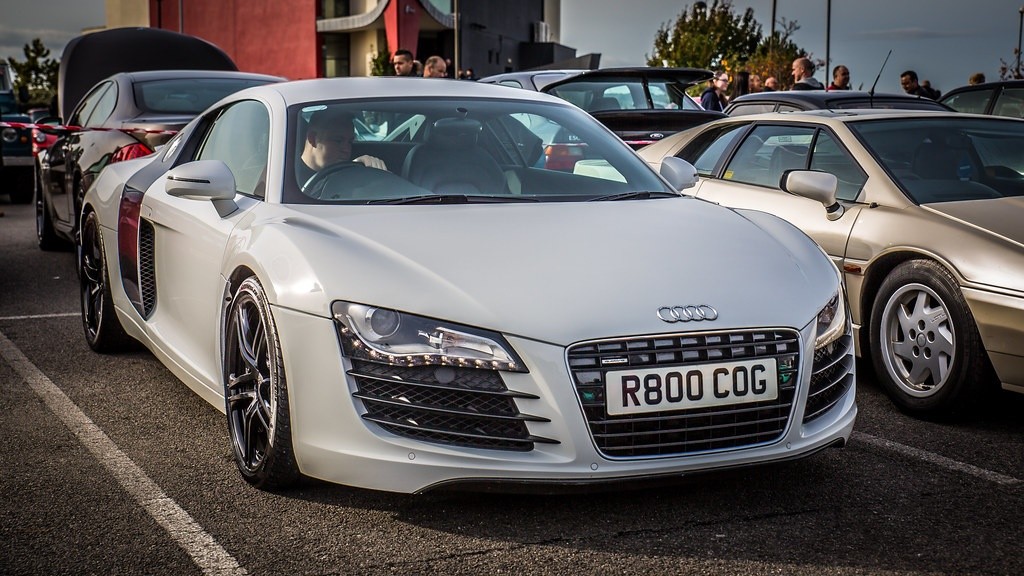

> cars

[
  {"left": 573, "top": 76, "right": 1024, "bottom": 426},
  {"left": 0, "top": 26, "right": 389, "bottom": 278},
  {"left": 382, "top": 67, "right": 732, "bottom": 161}
]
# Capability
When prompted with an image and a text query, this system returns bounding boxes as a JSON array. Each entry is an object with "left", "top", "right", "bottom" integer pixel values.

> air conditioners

[{"left": 534, "top": 21, "right": 551, "bottom": 43}]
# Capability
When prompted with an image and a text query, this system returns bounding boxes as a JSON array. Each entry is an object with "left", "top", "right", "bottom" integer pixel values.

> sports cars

[{"left": 75, "top": 73, "right": 859, "bottom": 501}]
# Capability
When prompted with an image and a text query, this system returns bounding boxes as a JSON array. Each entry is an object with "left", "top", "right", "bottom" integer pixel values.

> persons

[
  {"left": 900, "top": 71, "right": 936, "bottom": 99},
  {"left": 958, "top": 73, "right": 991, "bottom": 108},
  {"left": 699, "top": 71, "right": 730, "bottom": 113},
  {"left": 751, "top": 75, "right": 764, "bottom": 93},
  {"left": 727, "top": 71, "right": 754, "bottom": 107},
  {"left": 423, "top": 56, "right": 454, "bottom": 79},
  {"left": 764, "top": 76, "right": 778, "bottom": 92},
  {"left": 298, "top": 108, "right": 389, "bottom": 189},
  {"left": 393, "top": 48, "right": 418, "bottom": 76},
  {"left": 457, "top": 68, "right": 481, "bottom": 81},
  {"left": 922, "top": 80, "right": 941, "bottom": 99},
  {"left": 826, "top": 65, "right": 850, "bottom": 90},
  {"left": 410, "top": 60, "right": 423, "bottom": 77},
  {"left": 791, "top": 58, "right": 825, "bottom": 91}
]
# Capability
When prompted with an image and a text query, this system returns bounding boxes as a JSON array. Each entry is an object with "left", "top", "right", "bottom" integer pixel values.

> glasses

[{"left": 716, "top": 79, "right": 728, "bottom": 83}]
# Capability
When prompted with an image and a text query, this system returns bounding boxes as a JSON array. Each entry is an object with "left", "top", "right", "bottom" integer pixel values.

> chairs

[
  {"left": 914, "top": 140, "right": 982, "bottom": 182},
  {"left": 401, "top": 119, "right": 511, "bottom": 197},
  {"left": 769, "top": 144, "right": 817, "bottom": 189},
  {"left": 590, "top": 96, "right": 622, "bottom": 113}
]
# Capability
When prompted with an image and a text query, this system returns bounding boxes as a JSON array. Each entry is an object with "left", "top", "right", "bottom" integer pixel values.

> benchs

[{"left": 351, "top": 141, "right": 421, "bottom": 177}]
